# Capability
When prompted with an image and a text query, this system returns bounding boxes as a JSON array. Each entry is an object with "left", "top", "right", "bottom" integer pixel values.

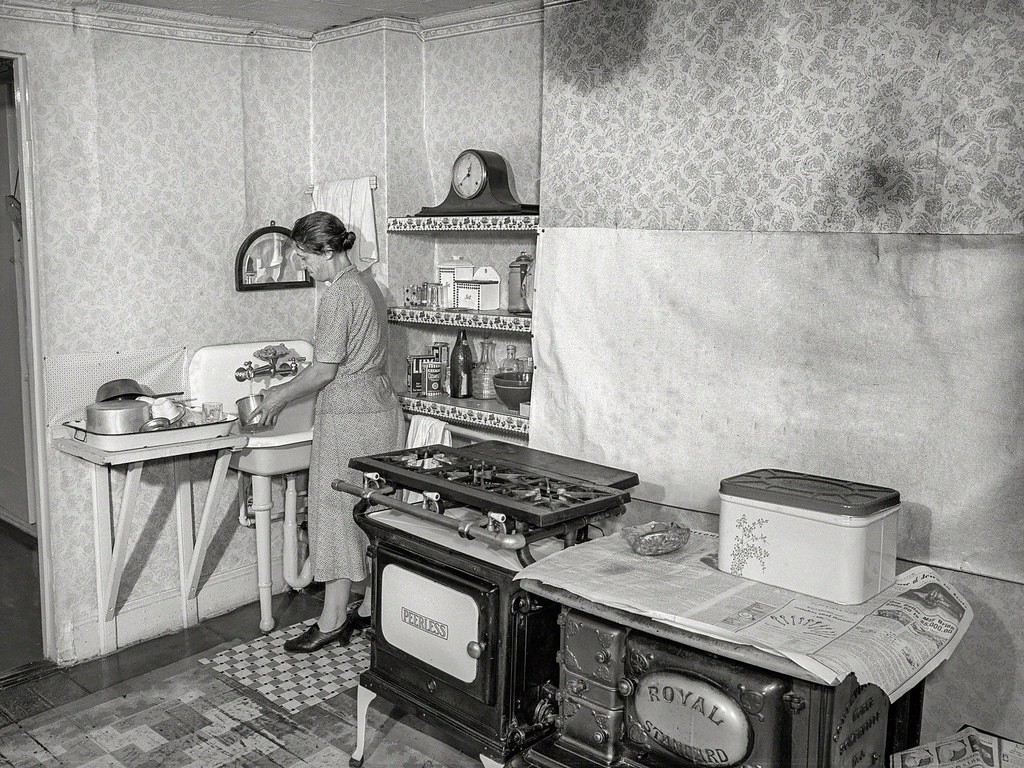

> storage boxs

[
  {"left": 719, "top": 468, "right": 901, "bottom": 605},
  {"left": 406, "top": 341, "right": 450, "bottom": 396},
  {"left": 455, "top": 280, "right": 499, "bottom": 310}
]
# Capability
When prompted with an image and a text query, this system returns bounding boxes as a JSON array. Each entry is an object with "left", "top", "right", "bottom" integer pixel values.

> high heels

[
  {"left": 283, "top": 614, "right": 356, "bottom": 654},
  {"left": 346, "top": 602, "right": 372, "bottom": 629}
]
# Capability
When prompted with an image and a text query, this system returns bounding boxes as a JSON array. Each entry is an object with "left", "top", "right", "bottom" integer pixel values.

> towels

[
  {"left": 401, "top": 415, "right": 454, "bottom": 510},
  {"left": 310, "top": 176, "right": 381, "bottom": 286}
]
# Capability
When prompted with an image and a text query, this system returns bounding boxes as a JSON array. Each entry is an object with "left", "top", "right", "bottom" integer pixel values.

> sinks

[{"left": 226, "top": 423, "right": 312, "bottom": 476}]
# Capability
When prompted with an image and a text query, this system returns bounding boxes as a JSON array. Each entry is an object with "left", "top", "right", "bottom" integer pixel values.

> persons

[{"left": 245, "top": 209, "right": 408, "bottom": 654}]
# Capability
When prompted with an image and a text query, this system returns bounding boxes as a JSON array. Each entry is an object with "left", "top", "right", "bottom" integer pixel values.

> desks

[{"left": 52, "top": 426, "right": 248, "bottom": 622}]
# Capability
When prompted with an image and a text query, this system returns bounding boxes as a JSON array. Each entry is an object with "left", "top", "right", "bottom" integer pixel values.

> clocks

[{"left": 414, "top": 149, "right": 540, "bottom": 216}]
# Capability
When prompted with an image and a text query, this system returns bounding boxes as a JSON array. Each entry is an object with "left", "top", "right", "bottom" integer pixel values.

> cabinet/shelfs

[{"left": 388, "top": 216, "right": 540, "bottom": 438}]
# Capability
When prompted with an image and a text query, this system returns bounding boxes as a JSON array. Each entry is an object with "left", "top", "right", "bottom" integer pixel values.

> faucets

[
  {"left": 280, "top": 356, "right": 301, "bottom": 378},
  {"left": 236, "top": 360, "right": 257, "bottom": 383}
]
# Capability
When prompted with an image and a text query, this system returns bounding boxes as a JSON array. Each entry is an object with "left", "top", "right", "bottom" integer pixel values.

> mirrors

[{"left": 235, "top": 220, "right": 313, "bottom": 292}]
